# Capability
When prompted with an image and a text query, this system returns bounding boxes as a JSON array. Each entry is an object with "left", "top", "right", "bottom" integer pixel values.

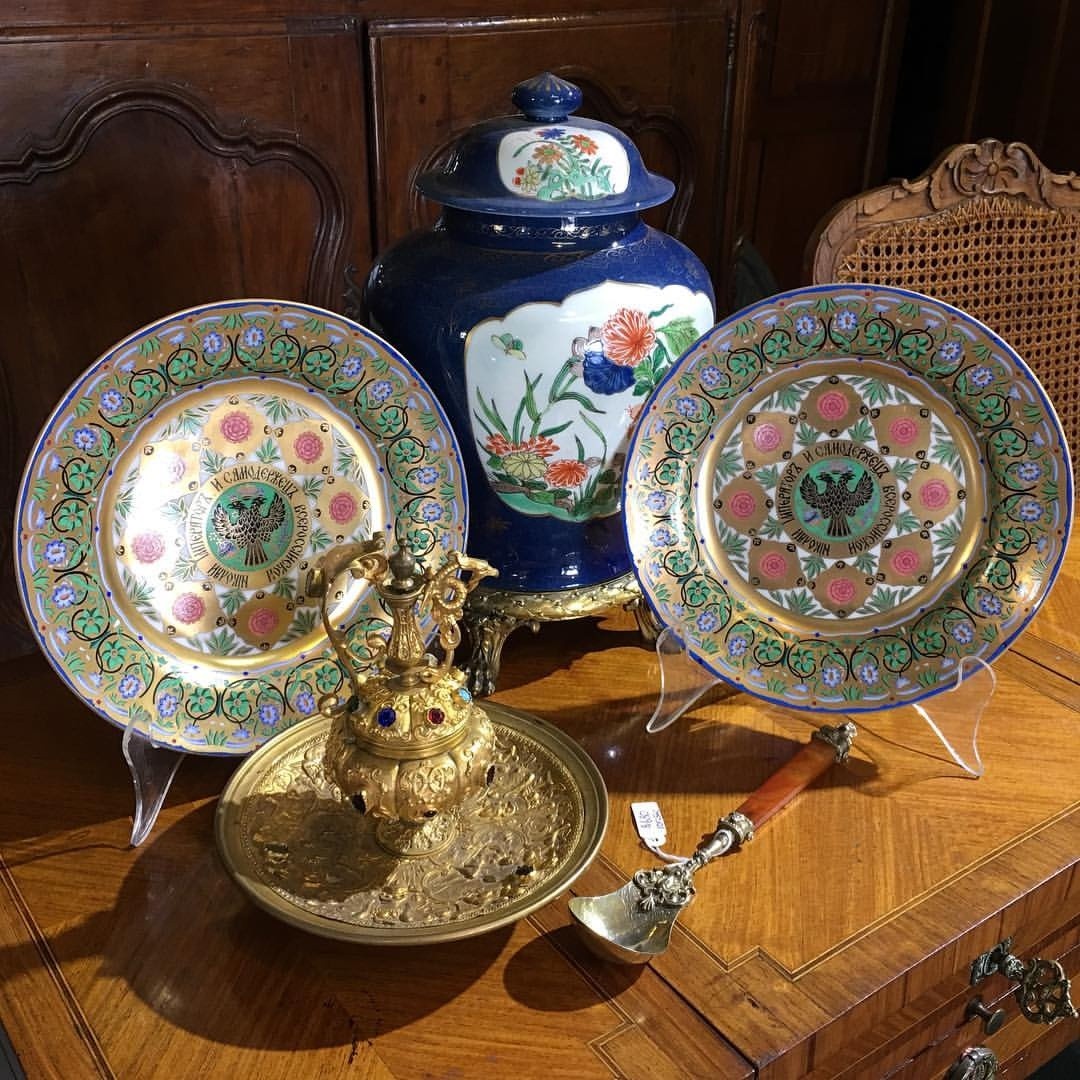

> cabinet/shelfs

[{"left": 0, "top": 0, "right": 1080, "bottom": 675}]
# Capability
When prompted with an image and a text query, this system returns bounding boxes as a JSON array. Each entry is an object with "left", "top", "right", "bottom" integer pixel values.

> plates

[
  {"left": 618, "top": 281, "right": 1075, "bottom": 706},
  {"left": 213, "top": 698, "right": 606, "bottom": 942},
  {"left": 10, "top": 296, "right": 471, "bottom": 756}
]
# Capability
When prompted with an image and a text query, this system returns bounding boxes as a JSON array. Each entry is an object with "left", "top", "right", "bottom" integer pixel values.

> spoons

[{"left": 566, "top": 720, "right": 858, "bottom": 966}]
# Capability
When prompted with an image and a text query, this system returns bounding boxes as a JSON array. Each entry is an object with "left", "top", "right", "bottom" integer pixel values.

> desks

[{"left": 0, "top": 499, "right": 1080, "bottom": 1080}]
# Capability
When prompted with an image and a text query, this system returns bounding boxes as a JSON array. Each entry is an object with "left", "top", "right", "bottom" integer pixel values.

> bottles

[{"left": 360, "top": 82, "right": 716, "bottom": 595}]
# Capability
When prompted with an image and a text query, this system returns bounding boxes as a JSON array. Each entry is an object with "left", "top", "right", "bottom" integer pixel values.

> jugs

[{"left": 320, "top": 533, "right": 500, "bottom": 857}]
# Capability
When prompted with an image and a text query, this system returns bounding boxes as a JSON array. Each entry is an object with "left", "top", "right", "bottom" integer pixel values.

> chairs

[{"left": 803, "top": 140, "right": 1080, "bottom": 503}]
